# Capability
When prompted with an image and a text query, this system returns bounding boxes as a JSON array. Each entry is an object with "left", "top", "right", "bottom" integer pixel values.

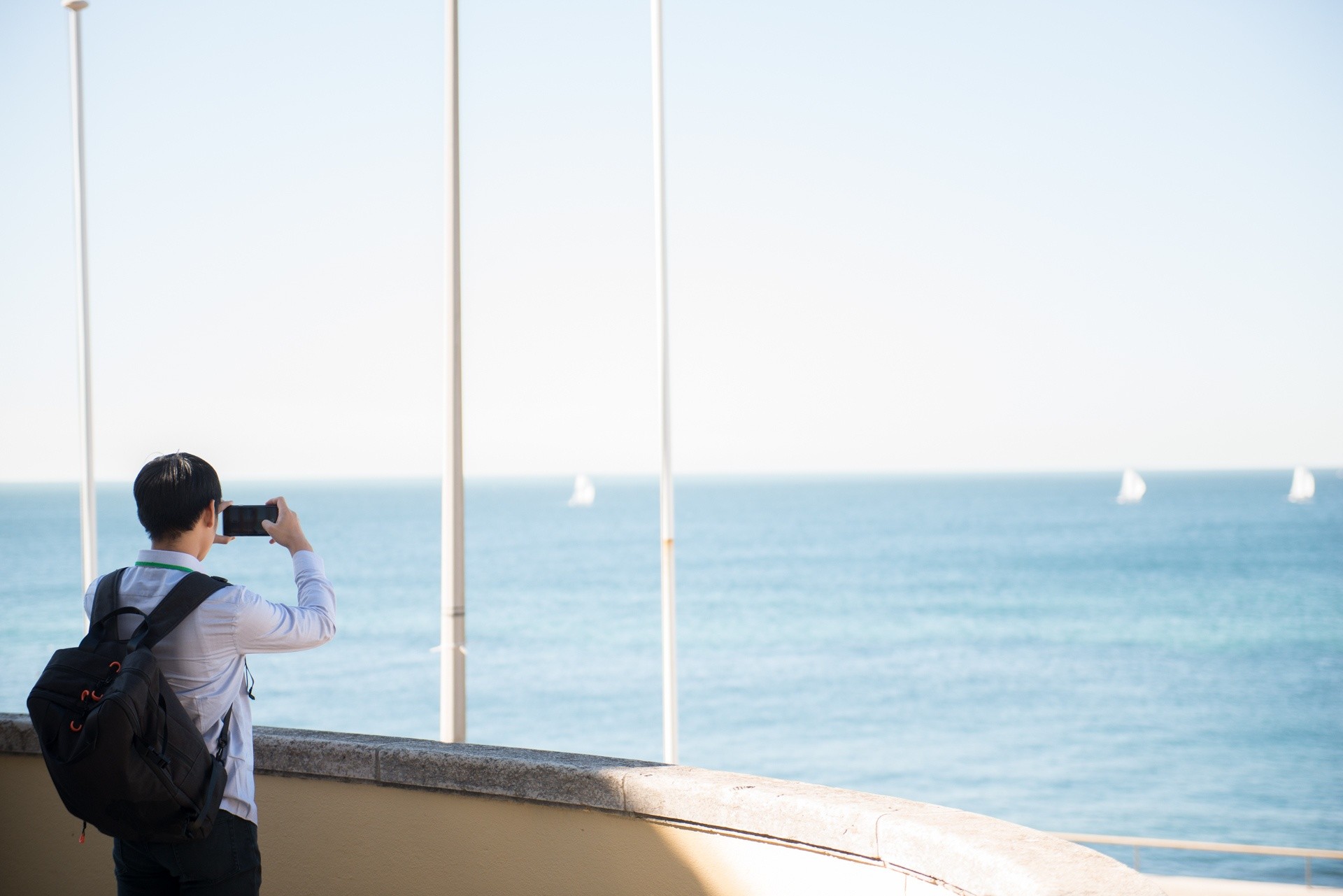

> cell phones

[{"left": 222, "top": 505, "right": 280, "bottom": 537}]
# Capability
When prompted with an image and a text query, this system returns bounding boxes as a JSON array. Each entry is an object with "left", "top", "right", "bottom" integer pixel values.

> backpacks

[{"left": 26, "top": 569, "right": 236, "bottom": 863}]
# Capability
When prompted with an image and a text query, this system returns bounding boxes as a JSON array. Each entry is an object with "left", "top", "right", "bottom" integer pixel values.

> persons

[{"left": 81, "top": 451, "right": 336, "bottom": 896}]
